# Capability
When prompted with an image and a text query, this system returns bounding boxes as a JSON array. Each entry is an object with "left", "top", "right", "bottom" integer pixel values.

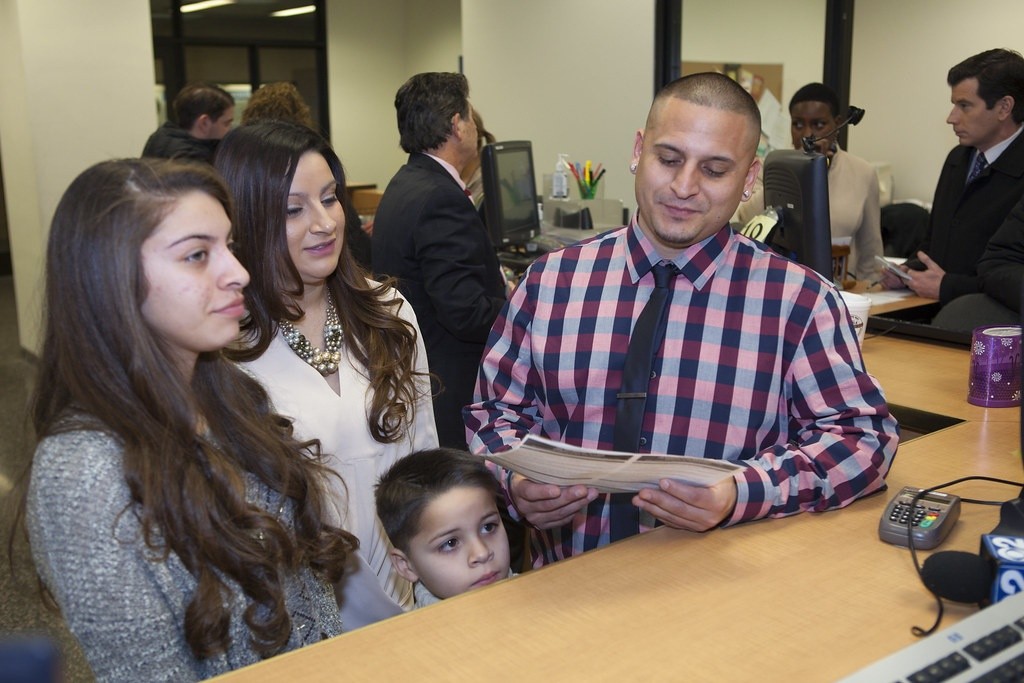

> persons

[
  {"left": 461, "top": 109, "right": 496, "bottom": 221},
  {"left": 375, "top": 445, "right": 522, "bottom": 610},
  {"left": 143, "top": 81, "right": 236, "bottom": 166},
  {"left": 931, "top": 196, "right": 1024, "bottom": 332},
  {"left": 214, "top": 116, "right": 441, "bottom": 615},
  {"left": 879, "top": 48, "right": 1024, "bottom": 325},
  {"left": 6, "top": 157, "right": 361, "bottom": 683},
  {"left": 461, "top": 70, "right": 899, "bottom": 570},
  {"left": 243, "top": 82, "right": 312, "bottom": 129},
  {"left": 789, "top": 83, "right": 884, "bottom": 281},
  {"left": 370, "top": 73, "right": 525, "bottom": 576}
]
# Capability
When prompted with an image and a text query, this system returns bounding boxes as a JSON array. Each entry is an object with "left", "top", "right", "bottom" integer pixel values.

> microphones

[{"left": 922, "top": 534, "right": 1024, "bottom": 608}]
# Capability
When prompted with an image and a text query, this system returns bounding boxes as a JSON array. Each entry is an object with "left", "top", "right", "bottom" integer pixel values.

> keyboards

[{"left": 840, "top": 590, "right": 1024, "bottom": 683}]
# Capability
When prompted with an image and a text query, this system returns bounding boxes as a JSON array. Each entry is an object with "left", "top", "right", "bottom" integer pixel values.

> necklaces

[{"left": 279, "top": 285, "right": 344, "bottom": 376}]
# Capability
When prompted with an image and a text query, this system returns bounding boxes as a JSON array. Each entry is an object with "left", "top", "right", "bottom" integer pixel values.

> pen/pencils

[{"left": 568, "top": 159, "right": 606, "bottom": 199}]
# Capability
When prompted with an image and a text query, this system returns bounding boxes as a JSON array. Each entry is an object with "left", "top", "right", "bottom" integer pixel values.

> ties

[
  {"left": 611, "top": 264, "right": 682, "bottom": 454},
  {"left": 965, "top": 152, "right": 988, "bottom": 185}
]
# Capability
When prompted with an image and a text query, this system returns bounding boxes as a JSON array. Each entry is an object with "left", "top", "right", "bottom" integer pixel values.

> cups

[
  {"left": 968, "top": 322, "right": 1023, "bottom": 408},
  {"left": 578, "top": 178, "right": 597, "bottom": 200},
  {"left": 839, "top": 290, "right": 872, "bottom": 351}
]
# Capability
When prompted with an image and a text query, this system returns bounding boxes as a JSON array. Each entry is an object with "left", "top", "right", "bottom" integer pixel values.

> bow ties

[{"left": 464, "top": 189, "right": 472, "bottom": 198}]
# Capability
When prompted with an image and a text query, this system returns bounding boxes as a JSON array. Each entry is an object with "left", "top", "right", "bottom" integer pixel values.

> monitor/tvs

[
  {"left": 479, "top": 139, "right": 541, "bottom": 268},
  {"left": 739, "top": 148, "right": 833, "bottom": 284}
]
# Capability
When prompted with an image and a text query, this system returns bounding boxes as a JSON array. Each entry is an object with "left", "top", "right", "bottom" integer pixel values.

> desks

[
  {"left": 205, "top": 330, "right": 1024, "bottom": 683},
  {"left": 840, "top": 271, "right": 939, "bottom": 315}
]
